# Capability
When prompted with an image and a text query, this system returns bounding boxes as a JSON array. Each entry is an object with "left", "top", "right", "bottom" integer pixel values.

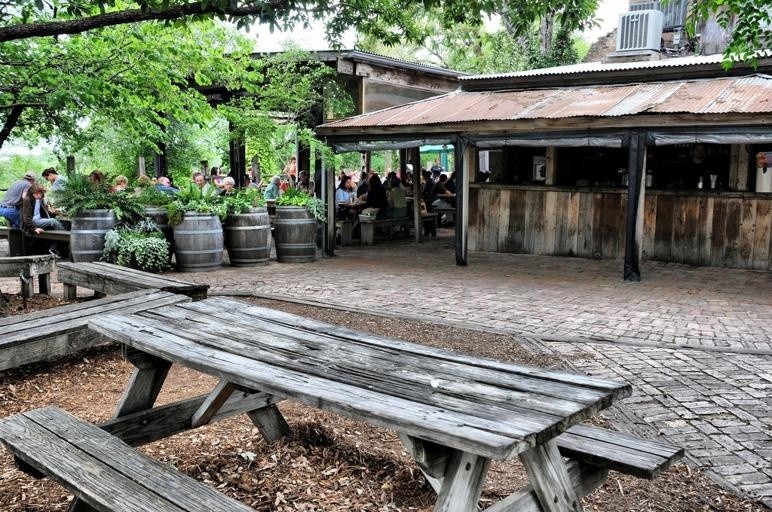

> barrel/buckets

[
  {"left": 173, "top": 211, "right": 224, "bottom": 271},
  {"left": 225, "top": 207, "right": 274, "bottom": 269},
  {"left": 70, "top": 208, "right": 114, "bottom": 262},
  {"left": 274, "top": 205, "right": 320, "bottom": 263},
  {"left": 136, "top": 204, "right": 174, "bottom": 271}
]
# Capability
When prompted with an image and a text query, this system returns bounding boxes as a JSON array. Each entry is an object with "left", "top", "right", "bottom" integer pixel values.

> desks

[{"left": 67, "top": 296, "right": 631, "bottom": 512}]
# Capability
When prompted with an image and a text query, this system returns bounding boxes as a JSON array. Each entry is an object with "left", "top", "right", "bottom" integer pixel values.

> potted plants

[{"left": 47, "top": 167, "right": 331, "bottom": 272}]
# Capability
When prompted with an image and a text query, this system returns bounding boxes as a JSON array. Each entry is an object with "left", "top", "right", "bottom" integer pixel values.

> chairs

[
  {"left": 3, "top": 402, "right": 258, "bottom": 511},
  {"left": 547, "top": 421, "right": 686, "bottom": 512}
]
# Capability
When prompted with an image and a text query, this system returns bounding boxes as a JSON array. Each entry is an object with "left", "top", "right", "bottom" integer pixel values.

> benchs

[
  {"left": 0, "top": 225, "right": 212, "bottom": 376},
  {"left": 334, "top": 206, "right": 457, "bottom": 246}
]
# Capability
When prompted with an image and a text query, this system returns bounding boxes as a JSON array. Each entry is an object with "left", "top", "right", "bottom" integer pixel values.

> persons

[
  {"left": 246, "top": 170, "right": 315, "bottom": 200},
  {"left": 336, "top": 170, "right": 457, "bottom": 221},
  {"left": 43, "top": 167, "right": 236, "bottom": 206},
  {"left": 0, "top": 170, "right": 65, "bottom": 257},
  {"left": 675, "top": 144, "right": 711, "bottom": 188}
]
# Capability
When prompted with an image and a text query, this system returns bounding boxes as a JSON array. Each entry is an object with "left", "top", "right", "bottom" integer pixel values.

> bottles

[
  {"left": 47, "top": 204, "right": 52, "bottom": 214},
  {"left": 696, "top": 175, "right": 704, "bottom": 192}
]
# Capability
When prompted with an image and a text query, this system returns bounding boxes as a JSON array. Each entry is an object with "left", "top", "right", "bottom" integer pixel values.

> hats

[
  {"left": 30, "top": 183, "right": 48, "bottom": 193},
  {"left": 23, "top": 171, "right": 36, "bottom": 179}
]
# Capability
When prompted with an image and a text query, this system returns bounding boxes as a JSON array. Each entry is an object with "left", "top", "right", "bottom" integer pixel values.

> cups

[{"left": 710, "top": 175, "right": 717, "bottom": 189}]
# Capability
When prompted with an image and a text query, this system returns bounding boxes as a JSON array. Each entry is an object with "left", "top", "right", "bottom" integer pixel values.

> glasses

[{"left": 45, "top": 176, "right": 48, "bottom": 180}]
